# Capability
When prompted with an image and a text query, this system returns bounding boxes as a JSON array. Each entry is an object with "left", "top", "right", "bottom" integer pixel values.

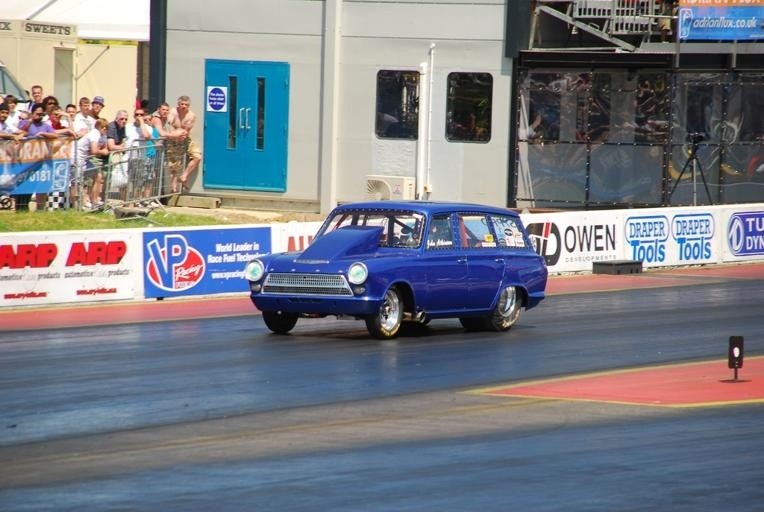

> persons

[
  {"left": 406, "top": 221, "right": 442, "bottom": 244},
  {"left": 0, "top": 85, "right": 202, "bottom": 213},
  {"left": 377, "top": 70, "right": 671, "bottom": 141}
]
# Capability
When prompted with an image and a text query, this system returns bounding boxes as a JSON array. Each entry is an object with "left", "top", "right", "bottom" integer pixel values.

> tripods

[{"left": 662, "top": 145, "right": 713, "bottom": 206}]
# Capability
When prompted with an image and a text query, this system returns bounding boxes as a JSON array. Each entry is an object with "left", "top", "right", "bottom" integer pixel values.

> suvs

[{"left": 243, "top": 198, "right": 550, "bottom": 338}]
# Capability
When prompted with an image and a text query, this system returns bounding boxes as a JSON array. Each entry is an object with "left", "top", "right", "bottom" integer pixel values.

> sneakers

[{"left": 84, "top": 199, "right": 163, "bottom": 213}]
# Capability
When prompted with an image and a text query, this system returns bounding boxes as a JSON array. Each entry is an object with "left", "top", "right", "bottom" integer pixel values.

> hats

[{"left": 91, "top": 96, "right": 105, "bottom": 107}]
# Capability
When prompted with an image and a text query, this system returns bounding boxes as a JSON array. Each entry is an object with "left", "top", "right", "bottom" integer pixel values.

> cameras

[{"left": 37, "top": 112, "right": 46, "bottom": 116}]
